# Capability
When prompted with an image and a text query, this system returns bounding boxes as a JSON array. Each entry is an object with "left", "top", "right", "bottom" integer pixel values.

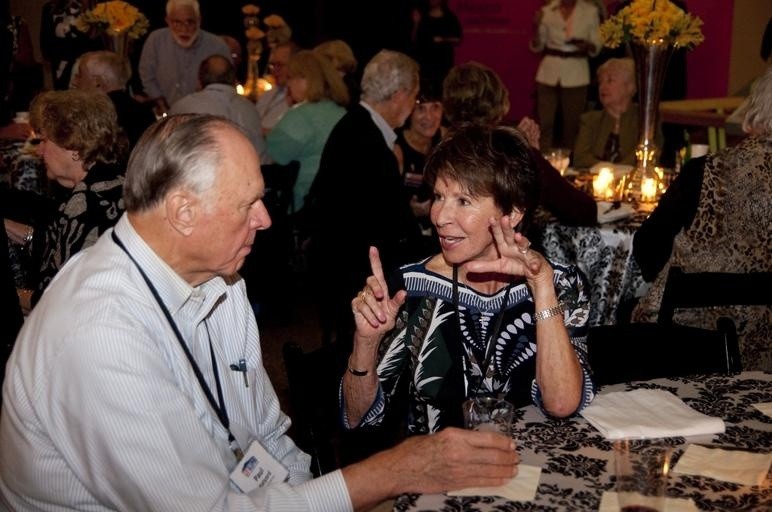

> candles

[
  {"left": 236, "top": 83, "right": 246, "bottom": 95},
  {"left": 643, "top": 177, "right": 656, "bottom": 197},
  {"left": 593, "top": 168, "right": 612, "bottom": 192}
]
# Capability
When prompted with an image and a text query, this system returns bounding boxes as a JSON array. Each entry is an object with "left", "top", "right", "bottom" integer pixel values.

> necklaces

[{"left": 457, "top": 269, "right": 506, "bottom": 329}]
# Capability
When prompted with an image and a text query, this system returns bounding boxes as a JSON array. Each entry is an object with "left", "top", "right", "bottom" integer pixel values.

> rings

[
  {"left": 361, "top": 290, "right": 374, "bottom": 302},
  {"left": 518, "top": 241, "right": 532, "bottom": 254}
]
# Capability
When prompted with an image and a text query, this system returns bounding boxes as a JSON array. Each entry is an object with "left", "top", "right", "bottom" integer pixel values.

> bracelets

[
  {"left": 17, "top": 289, "right": 31, "bottom": 296},
  {"left": 23, "top": 224, "right": 34, "bottom": 243},
  {"left": 345, "top": 352, "right": 378, "bottom": 377}
]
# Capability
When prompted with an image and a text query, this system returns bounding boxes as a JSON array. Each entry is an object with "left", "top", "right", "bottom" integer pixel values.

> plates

[{"left": 546, "top": 39, "right": 579, "bottom": 53}]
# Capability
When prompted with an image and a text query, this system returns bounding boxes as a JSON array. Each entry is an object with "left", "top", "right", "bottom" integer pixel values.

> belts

[{"left": 546, "top": 47, "right": 588, "bottom": 59}]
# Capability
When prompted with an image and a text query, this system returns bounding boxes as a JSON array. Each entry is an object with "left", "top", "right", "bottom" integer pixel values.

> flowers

[
  {"left": 82, "top": 0, "right": 145, "bottom": 39},
  {"left": 595, "top": 2, "right": 705, "bottom": 49}
]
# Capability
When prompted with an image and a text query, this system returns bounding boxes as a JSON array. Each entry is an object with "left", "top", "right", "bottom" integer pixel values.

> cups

[
  {"left": 612, "top": 437, "right": 674, "bottom": 512},
  {"left": 545, "top": 147, "right": 572, "bottom": 177},
  {"left": 462, "top": 397, "right": 514, "bottom": 436}
]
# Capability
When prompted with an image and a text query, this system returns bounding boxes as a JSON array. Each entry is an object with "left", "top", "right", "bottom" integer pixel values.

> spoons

[{"left": 602, "top": 200, "right": 622, "bottom": 215}]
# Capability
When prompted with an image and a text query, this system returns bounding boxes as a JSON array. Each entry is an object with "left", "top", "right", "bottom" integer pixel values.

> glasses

[
  {"left": 168, "top": 16, "right": 201, "bottom": 29},
  {"left": 405, "top": 87, "right": 426, "bottom": 105},
  {"left": 267, "top": 61, "right": 289, "bottom": 71}
]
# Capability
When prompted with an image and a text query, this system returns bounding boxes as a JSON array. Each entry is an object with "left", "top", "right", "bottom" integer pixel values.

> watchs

[{"left": 532, "top": 303, "right": 562, "bottom": 320}]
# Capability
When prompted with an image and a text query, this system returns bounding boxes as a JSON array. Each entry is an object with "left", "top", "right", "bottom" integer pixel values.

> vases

[
  {"left": 114, "top": 36, "right": 124, "bottom": 56},
  {"left": 631, "top": 46, "right": 664, "bottom": 195}
]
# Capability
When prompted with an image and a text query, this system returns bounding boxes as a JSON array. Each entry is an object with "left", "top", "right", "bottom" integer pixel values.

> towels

[
  {"left": 597, "top": 201, "right": 636, "bottom": 223},
  {"left": 580, "top": 388, "right": 724, "bottom": 441}
]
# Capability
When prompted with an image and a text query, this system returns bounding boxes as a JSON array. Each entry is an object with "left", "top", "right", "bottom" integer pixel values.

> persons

[
  {"left": 392, "top": 88, "right": 447, "bottom": 221},
  {"left": 1, "top": 88, "right": 131, "bottom": 310},
  {"left": 760, "top": 17, "right": 772, "bottom": 63},
  {"left": 6, "top": 90, "right": 60, "bottom": 291},
  {"left": 1, "top": 113, "right": 523, "bottom": 512},
  {"left": 297, "top": 49, "right": 427, "bottom": 475},
  {"left": 411, "top": 1, "right": 464, "bottom": 102},
  {"left": 632, "top": 64, "right": 772, "bottom": 375},
  {"left": 138, "top": 0, "right": 235, "bottom": 114},
  {"left": 421, "top": 60, "right": 597, "bottom": 258},
  {"left": 529, "top": 0, "right": 604, "bottom": 144},
  {"left": 572, "top": 56, "right": 666, "bottom": 172},
  {"left": 254, "top": 42, "right": 299, "bottom": 138},
  {"left": 261, "top": 49, "right": 349, "bottom": 245},
  {"left": 72, "top": 49, "right": 158, "bottom": 152},
  {"left": 155, "top": 53, "right": 267, "bottom": 157},
  {"left": 312, "top": 40, "right": 364, "bottom": 113},
  {"left": 336, "top": 123, "right": 602, "bottom": 433}
]
